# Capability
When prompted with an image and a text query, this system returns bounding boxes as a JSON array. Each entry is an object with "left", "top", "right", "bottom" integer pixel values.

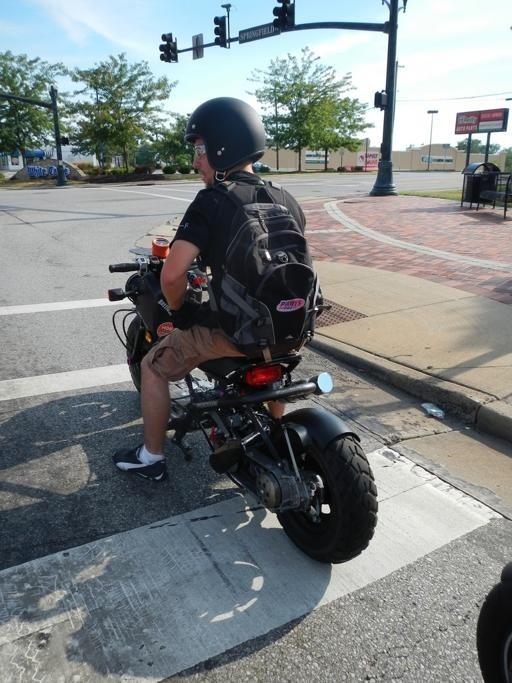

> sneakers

[{"left": 111, "top": 443, "right": 168, "bottom": 483}]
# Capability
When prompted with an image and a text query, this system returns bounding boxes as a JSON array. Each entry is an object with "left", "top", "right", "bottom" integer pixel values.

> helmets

[{"left": 183, "top": 97, "right": 266, "bottom": 172}]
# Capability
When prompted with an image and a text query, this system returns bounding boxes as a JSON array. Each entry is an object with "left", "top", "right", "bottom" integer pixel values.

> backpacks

[{"left": 206, "top": 180, "right": 324, "bottom": 369}]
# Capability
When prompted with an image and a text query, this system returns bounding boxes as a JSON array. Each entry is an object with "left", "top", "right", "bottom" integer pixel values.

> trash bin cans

[{"left": 461, "top": 162, "right": 500, "bottom": 211}]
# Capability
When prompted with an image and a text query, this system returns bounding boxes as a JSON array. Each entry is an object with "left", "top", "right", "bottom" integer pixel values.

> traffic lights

[
  {"left": 214, "top": 15, "right": 226, "bottom": 47},
  {"left": 158, "top": 34, "right": 178, "bottom": 64},
  {"left": 273, "top": 0, "right": 291, "bottom": 32}
]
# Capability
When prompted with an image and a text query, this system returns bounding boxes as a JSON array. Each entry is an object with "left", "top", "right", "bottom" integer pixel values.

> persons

[{"left": 111, "top": 95, "right": 316, "bottom": 483}]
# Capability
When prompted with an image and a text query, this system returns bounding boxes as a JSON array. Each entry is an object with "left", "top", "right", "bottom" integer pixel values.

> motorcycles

[
  {"left": 476, "top": 561, "right": 512, "bottom": 683},
  {"left": 108, "top": 235, "right": 378, "bottom": 566}
]
